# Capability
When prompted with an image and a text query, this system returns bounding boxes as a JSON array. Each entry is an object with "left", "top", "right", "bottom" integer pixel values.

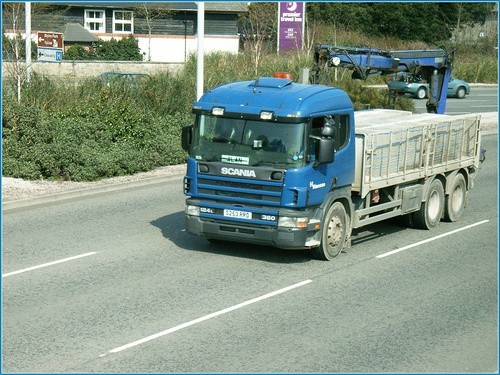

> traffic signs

[{"left": 37, "top": 31, "right": 64, "bottom": 62}]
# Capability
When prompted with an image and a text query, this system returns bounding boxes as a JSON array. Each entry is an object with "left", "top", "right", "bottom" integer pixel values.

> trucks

[{"left": 181, "top": 43, "right": 487, "bottom": 261}]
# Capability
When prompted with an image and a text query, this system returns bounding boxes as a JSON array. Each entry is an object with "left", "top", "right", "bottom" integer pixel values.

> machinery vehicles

[{"left": 391, "top": 74, "right": 470, "bottom": 98}]
